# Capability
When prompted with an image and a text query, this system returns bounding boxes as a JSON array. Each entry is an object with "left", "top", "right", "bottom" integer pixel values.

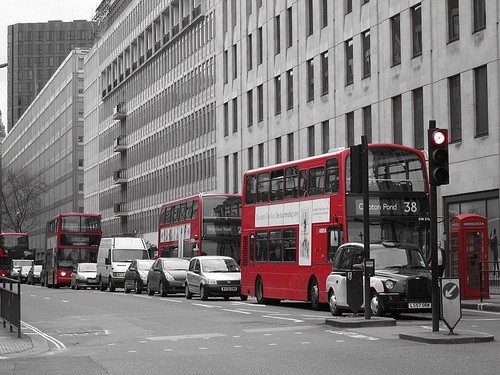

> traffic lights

[{"left": 427, "top": 128, "right": 450, "bottom": 185}]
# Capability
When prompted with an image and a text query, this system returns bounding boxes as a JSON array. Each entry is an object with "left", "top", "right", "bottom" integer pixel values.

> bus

[
  {"left": 240, "top": 143, "right": 431, "bottom": 310},
  {"left": 157, "top": 193, "right": 242, "bottom": 259},
  {"left": 40, "top": 212, "right": 102, "bottom": 288},
  {"left": 0, "top": 233, "right": 29, "bottom": 283}
]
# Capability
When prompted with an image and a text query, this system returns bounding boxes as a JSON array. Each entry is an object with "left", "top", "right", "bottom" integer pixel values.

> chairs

[{"left": 254, "top": 179, "right": 412, "bottom": 203}]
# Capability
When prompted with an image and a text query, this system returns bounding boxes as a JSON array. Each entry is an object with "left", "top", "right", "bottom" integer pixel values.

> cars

[
  {"left": 17, "top": 266, "right": 32, "bottom": 283},
  {"left": 71, "top": 263, "right": 98, "bottom": 290},
  {"left": 123, "top": 259, "right": 158, "bottom": 294},
  {"left": 27, "top": 265, "right": 44, "bottom": 285},
  {"left": 184, "top": 255, "right": 242, "bottom": 301},
  {"left": 325, "top": 242, "right": 441, "bottom": 317},
  {"left": 146, "top": 258, "right": 190, "bottom": 297}
]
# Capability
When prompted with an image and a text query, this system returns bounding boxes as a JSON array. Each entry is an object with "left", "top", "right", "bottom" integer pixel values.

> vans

[
  {"left": 96, "top": 237, "right": 150, "bottom": 292},
  {"left": 10, "top": 259, "right": 35, "bottom": 280}
]
